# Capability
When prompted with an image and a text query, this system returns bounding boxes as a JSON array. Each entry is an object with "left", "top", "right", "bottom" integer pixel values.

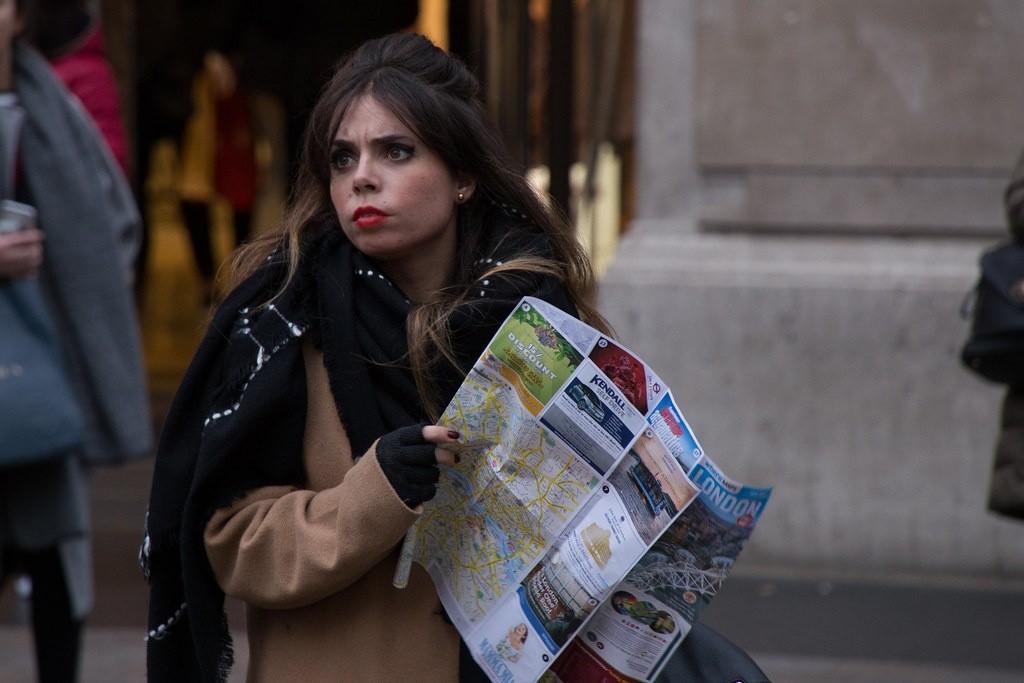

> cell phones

[{"left": 0, "top": 198, "right": 37, "bottom": 235}]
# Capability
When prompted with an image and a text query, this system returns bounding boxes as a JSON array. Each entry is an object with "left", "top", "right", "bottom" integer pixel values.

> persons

[
  {"left": 177, "top": 54, "right": 258, "bottom": 307},
  {"left": 0, "top": 0, "right": 153, "bottom": 683},
  {"left": 961, "top": 147, "right": 1024, "bottom": 519},
  {"left": 139, "top": 31, "right": 620, "bottom": 683}
]
